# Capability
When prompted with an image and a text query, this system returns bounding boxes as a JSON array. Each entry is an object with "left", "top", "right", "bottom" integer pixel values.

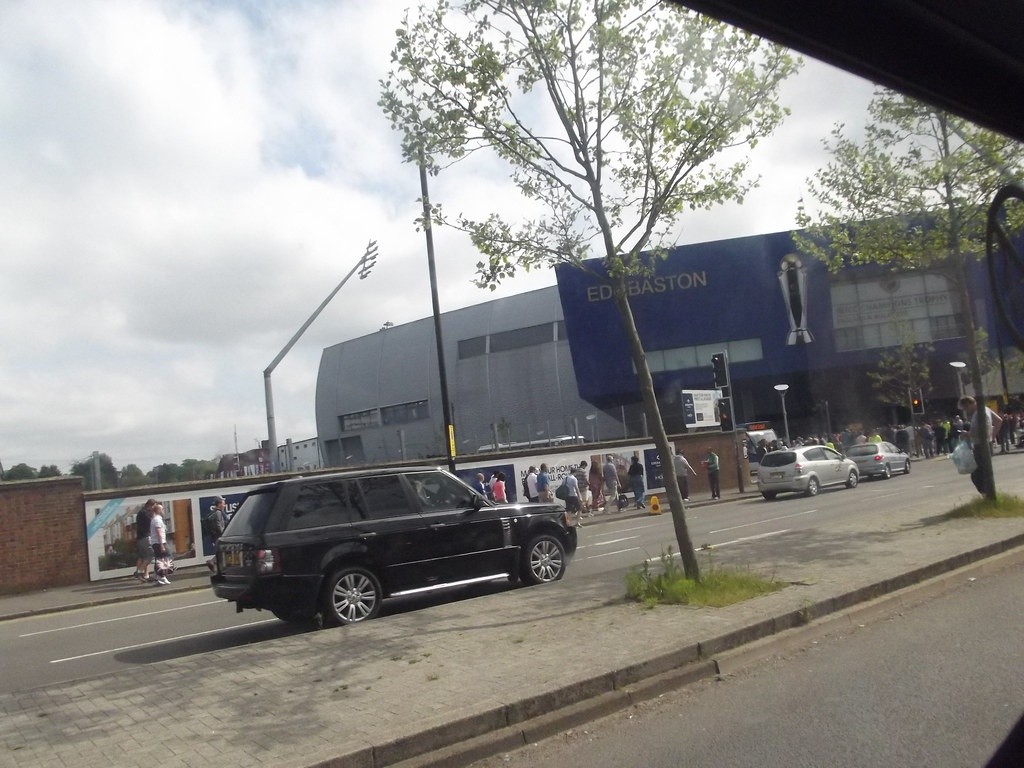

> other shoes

[
  {"left": 709, "top": 496, "right": 720, "bottom": 500},
  {"left": 575, "top": 522, "right": 582, "bottom": 529},
  {"left": 637, "top": 501, "right": 646, "bottom": 509},
  {"left": 157, "top": 577, "right": 171, "bottom": 585},
  {"left": 207, "top": 561, "right": 215, "bottom": 573}
]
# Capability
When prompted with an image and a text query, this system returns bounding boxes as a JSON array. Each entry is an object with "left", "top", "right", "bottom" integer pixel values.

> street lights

[
  {"left": 587, "top": 414, "right": 596, "bottom": 442},
  {"left": 773, "top": 384, "right": 791, "bottom": 448},
  {"left": 950, "top": 361, "right": 967, "bottom": 418}
]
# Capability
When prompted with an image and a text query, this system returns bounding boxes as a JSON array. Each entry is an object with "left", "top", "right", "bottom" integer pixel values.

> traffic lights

[
  {"left": 717, "top": 396, "right": 734, "bottom": 433},
  {"left": 711, "top": 351, "right": 729, "bottom": 388},
  {"left": 909, "top": 389, "right": 924, "bottom": 414}
]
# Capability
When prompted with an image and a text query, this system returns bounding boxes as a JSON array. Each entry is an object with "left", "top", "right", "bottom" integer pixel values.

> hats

[{"left": 215, "top": 496, "right": 225, "bottom": 501}]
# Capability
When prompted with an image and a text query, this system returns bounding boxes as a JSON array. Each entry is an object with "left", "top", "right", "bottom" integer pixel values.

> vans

[{"left": 736, "top": 421, "right": 778, "bottom": 471}]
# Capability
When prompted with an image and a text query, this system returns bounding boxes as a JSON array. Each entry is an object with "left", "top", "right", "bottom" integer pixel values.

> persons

[
  {"left": 576, "top": 461, "right": 595, "bottom": 520},
  {"left": 673, "top": 448, "right": 697, "bottom": 503},
  {"left": 700, "top": 446, "right": 721, "bottom": 501},
  {"left": 754, "top": 412, "right": 1024, "bottom": 466},
  {"left": 206, "top": 494, "right": 230, "bottom": 573},
  {"left": 627, "top": 455, "right": 647, "bottom": 509},
  {"left": 562, "top": 466, "right": 583, "bottom": 528},
  {"left": 956, "top": 396, "right": 1004, "bottom": 500},
  {"left": 133, "top": 498, "right": 174, "bottom": 585},
  {"left": 522, "top": 463, "right": 555, "bottom": 504},
  {"left": 474, "top": 470, "right": 509, "bottom": 504},
  {"left": 589, "top": 460, "right": 604, "bottom": 512},
  {"left": 603, "top": 455, "right": 626, "bottom": 515}
]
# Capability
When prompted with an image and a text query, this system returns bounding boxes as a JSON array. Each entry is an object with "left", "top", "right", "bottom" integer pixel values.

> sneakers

[
  {"left": 605, "top": 510, "right": 612, "bottom": 515},
  {"left": 618, "top": 508, "right": 626, "bottom": 512}
]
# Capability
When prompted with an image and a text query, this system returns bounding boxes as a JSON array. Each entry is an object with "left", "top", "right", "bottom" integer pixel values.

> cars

[
  {"left": 757, "top": 445, "right": 859, "bottom": 500},
  {"left": 846, "top": 441, "right": 912, "bottom": 479}
]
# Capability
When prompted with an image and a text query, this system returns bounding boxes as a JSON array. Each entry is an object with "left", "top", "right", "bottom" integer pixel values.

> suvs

[{"left": 211, "top": 465, "right": 578, "bottom": 631}]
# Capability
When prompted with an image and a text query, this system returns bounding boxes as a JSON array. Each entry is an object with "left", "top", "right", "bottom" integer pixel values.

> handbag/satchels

[
  {"left": 154, "top": 556, "right": 174, "bottom": 574},
  {"left": 953, "top": 436, "right": 978, "bottom": 474},
  {"left": 555, "top": 485, "right": 569, "bottom": 500},
  {"left": 619, "top": 495, "right": 629, "bottom": 507}
]
